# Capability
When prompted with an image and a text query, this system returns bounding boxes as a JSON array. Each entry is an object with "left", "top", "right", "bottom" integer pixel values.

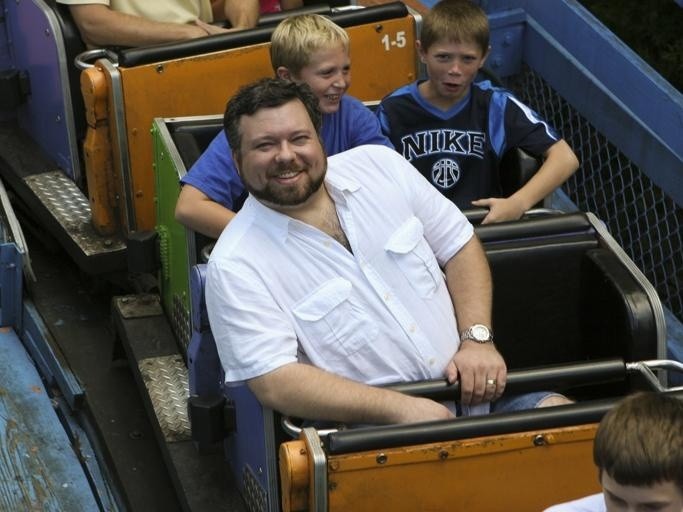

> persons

[
  {"left": 205, "top": 77, "right": 577, "bottom": 427},
  {"left": 543, "top": 391, "right": 683, "bottom": 512},
  {"left": 174, "top": 14, "right": 395, "bottom": 240},
  {"left": 56, "top": 0, "right": 259, "bottom": 64},
  {"left": 376, "top": 0, "right": 579, "bottom": 225}
]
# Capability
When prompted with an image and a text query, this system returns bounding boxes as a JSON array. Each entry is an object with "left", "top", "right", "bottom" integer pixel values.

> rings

[{"left": 486, "top": 379, "right": 497, "bottom": 384}]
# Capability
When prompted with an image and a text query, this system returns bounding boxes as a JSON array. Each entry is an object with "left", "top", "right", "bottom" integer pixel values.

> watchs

[{"left": 460, "top": 323, "right": 493, "bottom": 343}]
